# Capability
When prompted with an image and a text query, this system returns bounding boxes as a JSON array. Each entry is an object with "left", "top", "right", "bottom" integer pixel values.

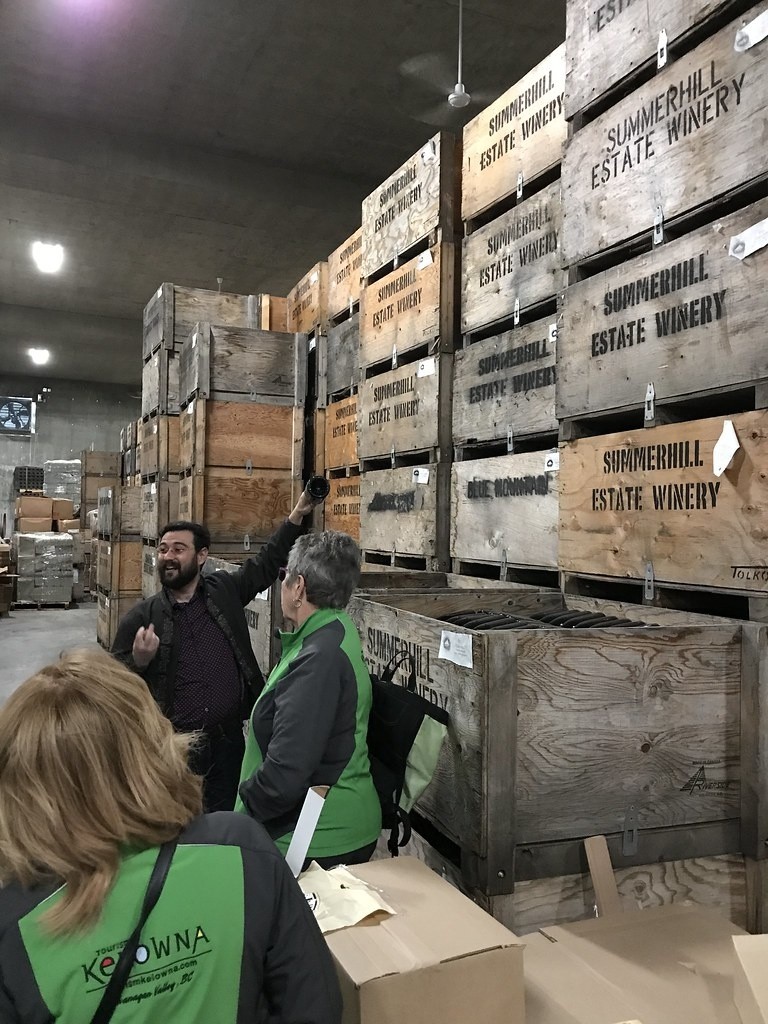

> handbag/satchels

[{"left": 365, "top": 651, "right": 449, "bottom": 858}]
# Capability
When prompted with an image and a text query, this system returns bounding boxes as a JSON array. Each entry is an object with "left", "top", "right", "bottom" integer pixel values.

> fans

[{"left": 397, "top": 0, "right": 515, "bottom": 126}]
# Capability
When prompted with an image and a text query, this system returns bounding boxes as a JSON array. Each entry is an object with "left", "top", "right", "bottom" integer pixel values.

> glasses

[
  {"left": 279, "top": 567, "right": 290, "bottom": 581},
  {"left": 155, "top": 544, "right": 198, "bottom": 554}
]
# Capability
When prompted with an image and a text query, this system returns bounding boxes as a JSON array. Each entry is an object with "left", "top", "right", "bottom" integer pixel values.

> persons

[
  {"left": 233, "top": 530, "right": 382, "bottom": 873},
  {"left": 0, "top": 647, "right": 343, "bottom": 1024},
  {"left": 112, "top": 475, "right": 330, "bottom": 813}
]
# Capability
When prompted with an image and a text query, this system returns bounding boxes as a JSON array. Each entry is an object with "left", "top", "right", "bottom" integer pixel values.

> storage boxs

[{"left": 10, "top": 0, "right": 768, "bottom": 1024}]
